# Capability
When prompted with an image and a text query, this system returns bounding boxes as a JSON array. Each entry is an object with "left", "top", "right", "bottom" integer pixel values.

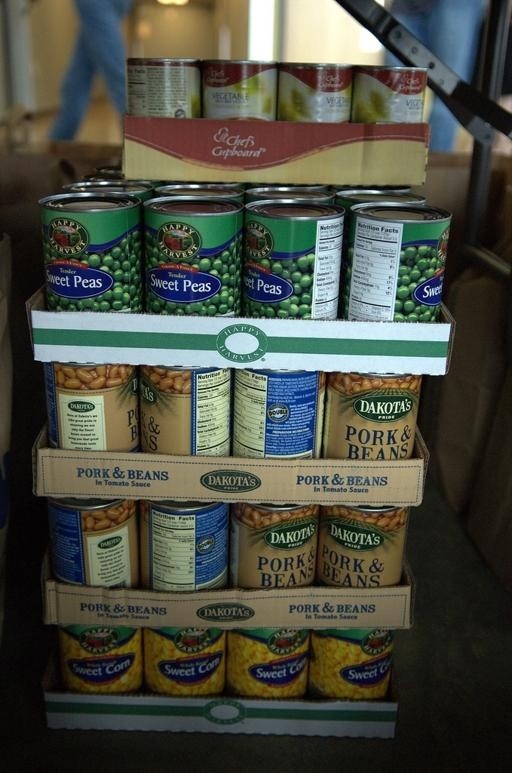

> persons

[
  {"left": 47, "top": 0, "right": 136, "bottom": 141},
  {"left": 381, "top": 0, "right": 489, "bottom": 152}
]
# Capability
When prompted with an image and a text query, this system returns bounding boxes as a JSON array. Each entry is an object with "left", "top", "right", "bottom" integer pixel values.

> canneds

[
  {"left": 50, "top": 496, "right": 410, "bottom": 592},
  {"left": 39, "top": 361, "right": 421, "bottom": 461},
  {"left": 126, "top": 58, "right": 428, "bottom": 125},
  {"left": 38, "top": 171, "right": 453, "bottom": 324},
  {"left": 60, "top": 620, "right": 397, "bottom": 702}
]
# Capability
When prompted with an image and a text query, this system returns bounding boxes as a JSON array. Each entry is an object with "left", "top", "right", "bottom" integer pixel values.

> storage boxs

[
  {"left": 462, "top": 361, "right": 512, "bottom": 595},
  {"left": 414, "top": 266, "right": 512, "bottom": 522}
]
